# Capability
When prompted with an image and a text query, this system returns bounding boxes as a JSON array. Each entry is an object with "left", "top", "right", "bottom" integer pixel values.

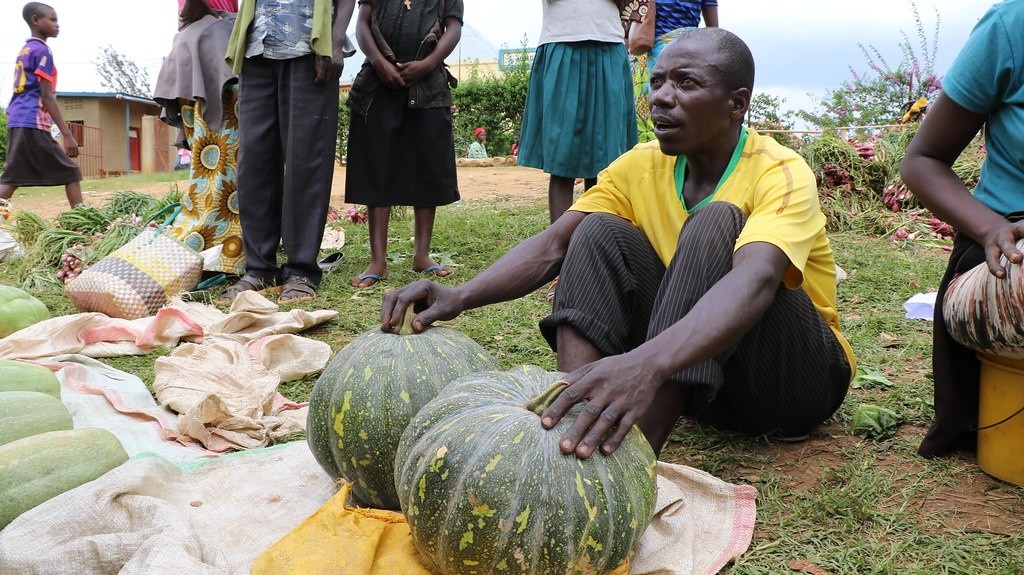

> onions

[
  {"left": 326, "top": 206, "right": 369, "bottom": 223},
  {"left": 55, "top": 213, "right": 174, "bottom": 284}
]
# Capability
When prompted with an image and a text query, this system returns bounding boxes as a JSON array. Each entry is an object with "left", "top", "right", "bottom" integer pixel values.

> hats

[{"left": 476, "top": 128, "right": 486, "bottom": 136}]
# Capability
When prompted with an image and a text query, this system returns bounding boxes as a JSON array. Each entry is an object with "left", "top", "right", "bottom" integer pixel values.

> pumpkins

[
  {"left": 307, "top": 303, "right": 504, "bottom": 511},
  {"left": 0, "top": 284, "right": 50, "bottom": 338},
  {"left": 0, "top": 358, "right": 128, "bottom": 532},
  {"left": 392, "top": 364, "right": 657, "bottom": 575}
]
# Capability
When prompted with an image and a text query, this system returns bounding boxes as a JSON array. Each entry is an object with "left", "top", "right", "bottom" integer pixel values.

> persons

[
  {"left": 468, "top": 128, "right": 488, "bottom": 157},
  {"left": 344, "top": 0, "right": 465, "bottom": 289},
  {"left": 0, "top": 2, "right": 84, "bottom": 210},
  {"left": 212, "top": 0, "right": 359, "bottom": 306},
  {"left": 378, "top": 27, "right": 857, "bottom": 464},
  {"left": 154, "top": 0, "right": 246, "bottom": 275},
  {"left": 899, "top": 0, "right": 1024, "bottom": 460},
  {"left": 648, "top": 0, "right": 718, "bottom": 76},
  {"left": 517, "top": 0, "right": 637, "bottom": 303}
]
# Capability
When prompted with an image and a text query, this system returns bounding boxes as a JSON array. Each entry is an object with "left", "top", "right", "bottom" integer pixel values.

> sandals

[
  {"left": 277, "top": 277, "right": 318, "bottom": 305},
  {"left": 218, "top": 275, "right": 282, "bottom": 307}
]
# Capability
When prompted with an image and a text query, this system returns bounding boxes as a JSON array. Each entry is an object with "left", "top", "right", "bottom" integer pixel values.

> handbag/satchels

[
  {"left": 629, "top": 0, "right": 656, "bottom": 56},
  {"left": 65, "top": 202, "right": 226, "bottom": 321}
]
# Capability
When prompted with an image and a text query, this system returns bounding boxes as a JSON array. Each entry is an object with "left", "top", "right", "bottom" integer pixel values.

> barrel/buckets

[{"left": 974, "top": 349, "right": 1024, "bottom": 488}]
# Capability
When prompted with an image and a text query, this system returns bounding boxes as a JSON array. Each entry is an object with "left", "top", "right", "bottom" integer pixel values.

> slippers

[
  {"left": 412, "top": 264, "right": 454, "bottom": 279},
  {"left": 352, "top": 274, "right": 386, "bottom": 290}
]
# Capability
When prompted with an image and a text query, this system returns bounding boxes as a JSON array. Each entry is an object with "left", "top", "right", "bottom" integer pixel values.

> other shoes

[
  {"left": 547, "top": 275, "right": 561, "bottom": 304},
  {"left": 317, "top": 252, "right": 345, "bottom": 272}
]
